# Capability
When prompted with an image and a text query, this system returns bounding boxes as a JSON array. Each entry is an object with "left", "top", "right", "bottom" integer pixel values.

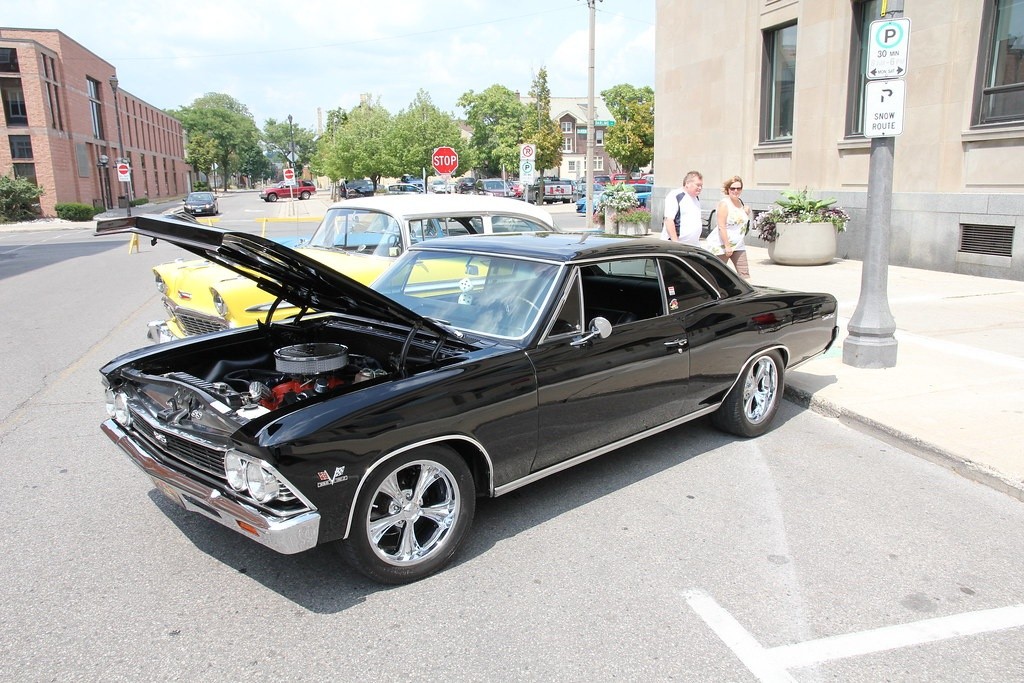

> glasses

[{"left": 728, "top": 187, "right": 742, "bottom": 191}]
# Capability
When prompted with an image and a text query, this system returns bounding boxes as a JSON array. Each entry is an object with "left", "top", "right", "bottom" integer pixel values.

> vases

[
  {"left": 605, "top": 206, "right": 648, "bottom": 235},
  {"left": 768, "top": 222, "right": 837, "bottom": 265}
]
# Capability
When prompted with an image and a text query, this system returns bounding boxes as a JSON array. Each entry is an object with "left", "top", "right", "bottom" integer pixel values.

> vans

[
  {"left": 386, "top": 183, "right": 436, "bottom": 195},
  {"left": 339, "top": 179, "right": 374, "bottom": 200},
  {"left": 571, "top": 176, "right": 611, "bottom": 203}
]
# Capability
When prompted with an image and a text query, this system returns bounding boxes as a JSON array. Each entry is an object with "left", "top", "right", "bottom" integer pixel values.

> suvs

[
  {"left": 399, "top": 175, "right": 424, "bottom": 192},
  {"left": 453, "top": 177, "right": 476, "bottom": 194},
  {"left": 473, "top": 177, "right": 516, "bottom": 198},
  {"left": 259, "top": 178, "right": 316, "bottom": 202},
  {"left": 429, "top": 180, "right": 451, "bottom": 194}
]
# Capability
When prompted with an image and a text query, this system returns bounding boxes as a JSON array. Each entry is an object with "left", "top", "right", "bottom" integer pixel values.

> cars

[
  {"left": 145, "top": 198, "right": 560, "bottom": 347},
  {"left": 181, "top": 190, "right": 218, "bottom": 216},
  {"left": 99, "top": 214, "right": 838, "bottom": 587},
  {"left": 575, "top": 182, "right": 653, "bottom": 214}
]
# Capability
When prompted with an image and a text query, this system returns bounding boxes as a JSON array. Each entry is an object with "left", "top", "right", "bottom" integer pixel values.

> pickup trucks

[{"left": 526, "top": 175, "right": 574, "bottom": 205}]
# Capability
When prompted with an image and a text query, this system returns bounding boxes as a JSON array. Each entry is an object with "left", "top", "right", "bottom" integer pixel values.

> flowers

[
  {"left": 750, "top": 188, "right": 851, "bottom": 242},
  {"left": 593, "top": 181, "right": 651, "bottom": 224}
]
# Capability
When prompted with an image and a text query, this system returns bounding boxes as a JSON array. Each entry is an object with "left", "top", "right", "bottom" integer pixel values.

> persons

[
  {"left": 701, "top": 175, "right": 753, "bottom": 285},
  {"left": 660, "top": 171, "right": 703, "bottom": 247}
]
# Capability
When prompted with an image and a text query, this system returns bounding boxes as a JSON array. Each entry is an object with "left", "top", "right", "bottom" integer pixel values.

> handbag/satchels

[{"left": 737, "top": 198, "right": 750, "bottom": 236}]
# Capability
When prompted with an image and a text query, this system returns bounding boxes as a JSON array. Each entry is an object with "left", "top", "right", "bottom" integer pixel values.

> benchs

[
  {"left": 578, "top": 275, "right": 664, "bottom": 327},
  {"left": 699, "top": 210, "right": 717, "bottom": 238}
]
